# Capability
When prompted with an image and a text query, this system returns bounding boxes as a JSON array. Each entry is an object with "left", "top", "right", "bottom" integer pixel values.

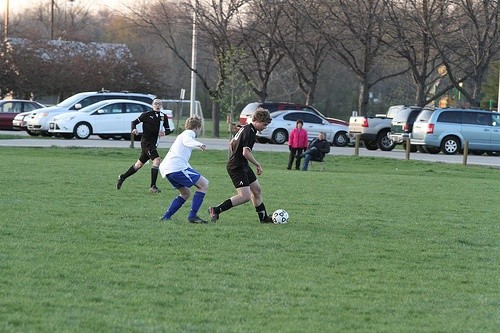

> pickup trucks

[{"left": 348, "top": 104, "right": 413, "bottom": 151}]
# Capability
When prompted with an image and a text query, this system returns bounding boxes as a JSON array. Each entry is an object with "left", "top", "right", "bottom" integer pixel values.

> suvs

[
  {"left": 386, "top": 103, "right": 437, "bottom": 154},
  {"left": 423, "top": 105, "right": 500, "bottom": 155},
  {"left": 26, "top": 87, "right": 164, "bottom": 137},
  {"left": 231, "top": 101, "right": 349, "bottom": 135}
]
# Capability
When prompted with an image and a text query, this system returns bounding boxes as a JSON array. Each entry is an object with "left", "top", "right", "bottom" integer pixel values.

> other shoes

[
  {"left": 161, "top": 216, "right": 171, "bottom": 220},
  {"left": 260, "top": 214, "right": 273, "bottom": 223},
  {"left": 294, "top": 154, "right": 301, "bottom": 158},
  {"left": 187, "top": 215, "right": 208, "bottom": 224},
  {"left": 296, "top": 167, "right": 300, "bottom": 170},
  {"left": 302, "top": 168, "right": 308, "bottom": 171},
  {"left": 287, "top": 167, "right": 291, "bottom": 170},
  {"left": 117, "top": 173, "right": 123, "bottom": 190},
  {"left": 208, "top": 206, "right": 219, "bottom": 223},
  {"left": 150, "top": 185, "right": 161, "bottom": 193}
]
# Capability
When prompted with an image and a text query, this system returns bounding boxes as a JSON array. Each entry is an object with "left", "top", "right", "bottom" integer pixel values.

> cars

[
  {"left": 48, "top": 99, "right": 176, "bottom": 140},
  {"left": 255, "top": 108, "right": 349, "bottom": 147},
  {"left": 0, "top": 99, "right": 47, "bottom": 130},
  {"left": 12, "top": 107, "right": 48, "bottom": 136}
]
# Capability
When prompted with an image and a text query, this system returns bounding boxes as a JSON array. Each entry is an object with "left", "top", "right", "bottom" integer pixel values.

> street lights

[{"left": 50, "top": 0, "right": 75, "bottom": 40}]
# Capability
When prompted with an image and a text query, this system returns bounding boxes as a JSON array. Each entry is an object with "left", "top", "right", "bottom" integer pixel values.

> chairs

[{"left": 310, "top": 152, "right": 325, "bottom": 171}]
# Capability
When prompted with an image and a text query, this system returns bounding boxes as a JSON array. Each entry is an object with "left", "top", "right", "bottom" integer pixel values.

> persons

[
  {"left": 116, "top": 97, "right": 170, "bottom": 193},
  {"left": 294, "top": 132, "right": 330, "bottom": 171},
  {"left": 159, "top": 117, "right": 209, "bottom": 223},
  {"left": 208, "top": 108, "right": 273, "bottom": 223},
  {"left": 287, "top": 120, "right": 308, "bottom": 170},
  {"left": 0, "top": 90, "right": 16, "bottom": 113}
]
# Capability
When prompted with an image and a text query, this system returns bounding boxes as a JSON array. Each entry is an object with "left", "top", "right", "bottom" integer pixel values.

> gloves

[
  {"left": 304, "top": 147, "right": 307, "bottom": 152},
  {"left": 289, "top": 145, "right": 292, "bottom": 151}
]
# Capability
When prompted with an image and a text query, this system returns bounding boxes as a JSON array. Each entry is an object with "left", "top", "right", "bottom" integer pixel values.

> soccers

[{"left": 272, "top": 209, "right": 289, "bottom": 224}]
